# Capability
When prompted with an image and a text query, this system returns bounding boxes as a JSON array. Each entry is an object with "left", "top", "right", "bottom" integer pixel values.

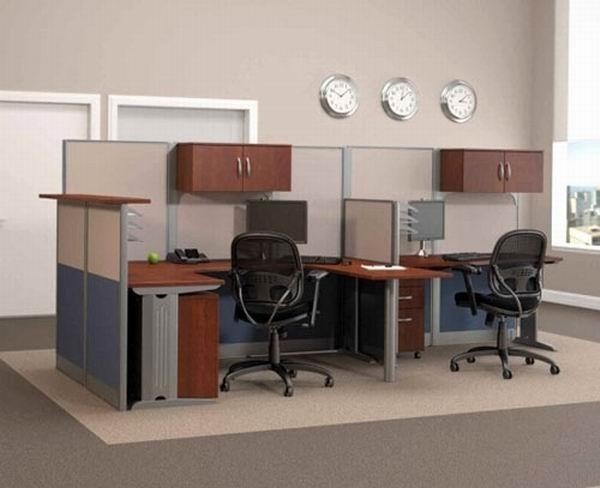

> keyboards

[
  {"left": 278, "top": 253, "right": 342, "bottom": 266},
  {"left": 443, "top": 252, "right": 492, "bottom": 261}
]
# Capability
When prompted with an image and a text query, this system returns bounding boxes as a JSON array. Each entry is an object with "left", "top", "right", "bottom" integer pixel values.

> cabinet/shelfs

[
  {"left": 440, "top": 149, "right": 543, "bottom": 193},
  {"left": 177, "top": 143, "right": 292, "bottom": 192},
  {"left": 398, "top": 280, "right": 425, "bottom": 358}
]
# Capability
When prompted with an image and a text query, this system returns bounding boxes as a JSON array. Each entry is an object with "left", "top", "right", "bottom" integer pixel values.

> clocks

[
  {"left": 319, "top": 73, "right": 360, "bottom": 118},
  {"left": 380, "top": 77, "right": 421, "bottom": 121},
  {"left": 440, "top": 80, "right": 477, "bottom": 124}
]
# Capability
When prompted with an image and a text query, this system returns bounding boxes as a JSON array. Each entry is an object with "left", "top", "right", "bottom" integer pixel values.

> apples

[{"left": 148, "top": 253, "right": 160, "bottom": 264}]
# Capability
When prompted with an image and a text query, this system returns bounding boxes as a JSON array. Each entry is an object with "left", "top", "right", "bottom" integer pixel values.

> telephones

[{"left": 167, "top": 248, "right": 210, "bottom": 264}]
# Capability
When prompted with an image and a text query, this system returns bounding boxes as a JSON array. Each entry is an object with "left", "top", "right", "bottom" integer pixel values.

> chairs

[
  {"left": 450, "top": 229, "right": 560, "bottom": 380},
  {"left": 220, "top": 230, "right": 337, "bottom": 397}
]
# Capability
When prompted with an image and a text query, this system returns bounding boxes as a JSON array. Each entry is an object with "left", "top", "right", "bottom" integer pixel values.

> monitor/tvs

[
  {"left": 245, "top": 199, "right": 309, "bottom": 244},
  {"left": 407, "top": 200, "right": 446, "bottom": 240}
]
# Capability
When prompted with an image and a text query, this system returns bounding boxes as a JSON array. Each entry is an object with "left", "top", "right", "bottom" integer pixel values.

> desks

[
  {"left": 400, "top": 254, "right": 562, "bottom": 352},
  {"left": 129, "top": 259, "right": 453, "bottom": 401}
]
